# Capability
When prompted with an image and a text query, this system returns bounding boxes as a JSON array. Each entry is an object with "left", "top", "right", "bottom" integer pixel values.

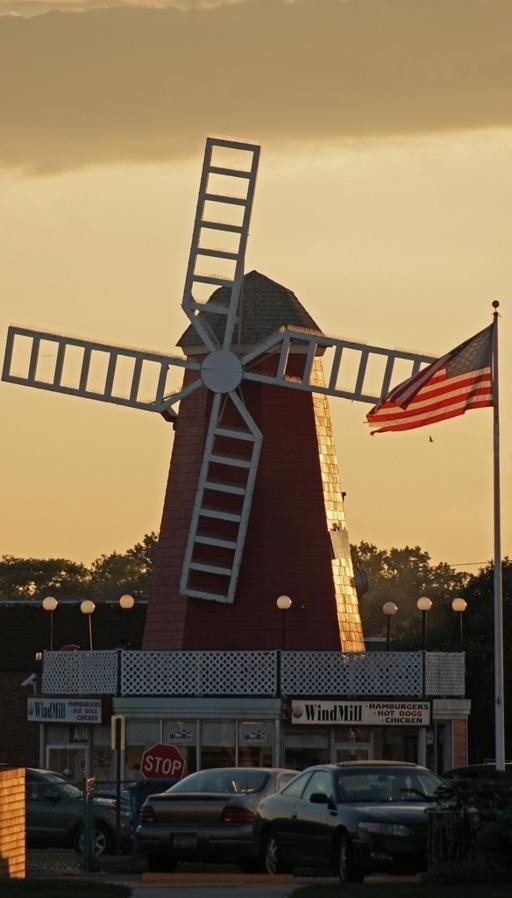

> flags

[{"left": 365, "top": 325, "right": 494, "bottom": 433}]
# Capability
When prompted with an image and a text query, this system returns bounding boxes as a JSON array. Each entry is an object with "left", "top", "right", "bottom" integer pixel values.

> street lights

[
  {"left": 276, "top": 593, "right": 295, "bottom": 651},
  {"left": 79, "top": 599, "right": 97, "bottom": 653},
  {"left": 119, "top": 592, "right": 136, "bottom": 651},
  {"left": 450, "top": 597, "right": 469, "bottom": 651},
  {"left": 42, "top": 596, "right": 59, "bottom": 651},
  {"left": 381, "top": 600, "right": 399, "bottom": 650},
  {"left": 416, "top": 595, "right": 433, "bottom": 652}
]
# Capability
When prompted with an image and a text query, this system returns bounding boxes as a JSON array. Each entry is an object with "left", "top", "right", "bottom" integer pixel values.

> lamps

[
  {"left": 42, "top": 594, "right": 135, "bottom": 649},
  {"left": 277, "top": 594, "right": 293, "bottom": 649},
  {"left": 383, "top": 596, "right": 467, "bottom": 651}
]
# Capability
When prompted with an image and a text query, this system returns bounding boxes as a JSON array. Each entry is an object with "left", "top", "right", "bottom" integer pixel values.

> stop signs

[{"left": 139, "top": 743, "right": 187, "bottom": 780}]
